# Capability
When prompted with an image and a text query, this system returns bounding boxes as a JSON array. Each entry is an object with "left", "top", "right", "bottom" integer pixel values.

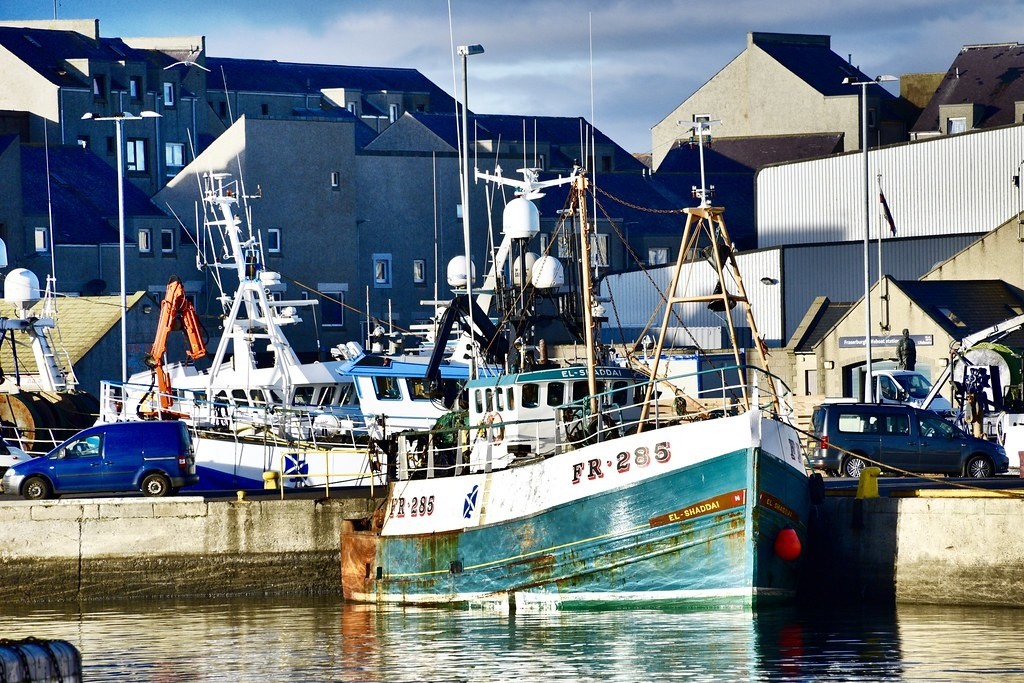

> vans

[
  {"left": 2, "top": 420, "right": 201, "bottom": 501},
  {"left": 802, "top": 401, "right": 1009, "bottom": 479}
]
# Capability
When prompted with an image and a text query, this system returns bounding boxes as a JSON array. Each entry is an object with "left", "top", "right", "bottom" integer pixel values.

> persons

[{"left": 896, "top": 329, "right": 916, "bottom": 372}]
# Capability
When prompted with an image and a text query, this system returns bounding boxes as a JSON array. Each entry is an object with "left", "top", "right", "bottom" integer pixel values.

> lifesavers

[{"left": 488, "top": 412, "right": 504, "bottom": 446}]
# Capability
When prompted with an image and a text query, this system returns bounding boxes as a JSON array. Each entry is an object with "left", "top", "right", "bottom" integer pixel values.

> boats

[
  {"left": 87, "top": 61, "right": 392, "bottom": 488},
  {"left": 337, "top": 5, "right": 826, "bottom": 633}
]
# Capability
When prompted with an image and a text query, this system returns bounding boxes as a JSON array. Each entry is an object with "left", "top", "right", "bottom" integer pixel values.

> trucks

[{"left": 864, "top": 369, "right": 955, "bottom": 434}]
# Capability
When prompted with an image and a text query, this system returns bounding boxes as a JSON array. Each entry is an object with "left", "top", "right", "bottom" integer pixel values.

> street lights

[
  {"left": 838, "top": 76, "right": 900, "bottom": 403},
  {"left": 82, "top": 111, "right": 162, "bottom": 384},
  {"left": 451, "top": 43, "right": 486, "bottom": 377}
]
